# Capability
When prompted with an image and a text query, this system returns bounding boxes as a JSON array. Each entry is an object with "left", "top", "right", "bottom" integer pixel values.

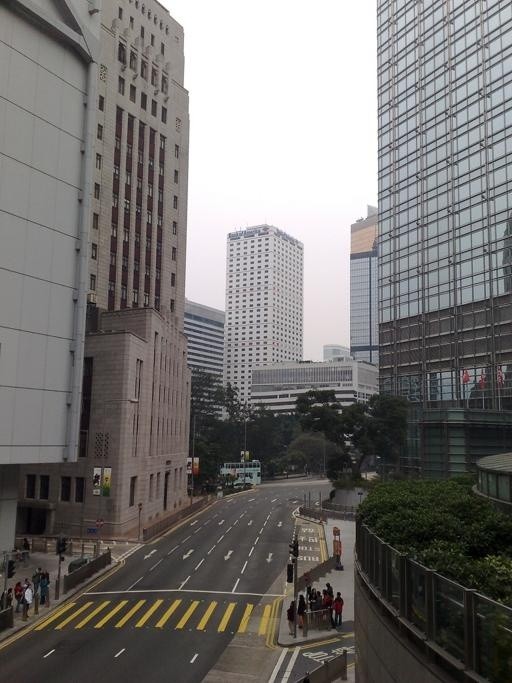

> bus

[{"left": 218, "top": 459, "right": 263, "bottom": 486}]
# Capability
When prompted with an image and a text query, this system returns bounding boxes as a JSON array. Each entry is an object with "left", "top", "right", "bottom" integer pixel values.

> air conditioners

[{"left": 86, "top": 292, "right": 98, "bottom": 304}]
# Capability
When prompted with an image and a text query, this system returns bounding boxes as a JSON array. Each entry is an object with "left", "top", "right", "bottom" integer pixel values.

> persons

[
  {"left": 286, "top": 583, "right": 344, "bottom": 635},
  {"left": 0, "top": 567, "right": 50, "bottom": 618},
  {"left": 23, "top": 538, "right": 30, "bottom": 558},
  {"left": 215, "top": 483, "right": 224, "bottom": 500}
]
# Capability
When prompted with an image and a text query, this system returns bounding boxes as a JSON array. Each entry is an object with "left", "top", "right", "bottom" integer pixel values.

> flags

[
  {"left": 462, "top": 368, "right": 470, "bottom": 382},
  {"left": 478, "top": 372, "right": 488, "bottom": 389},
  {"left": 498, "top": 368, "right": 504, "bottom": 387}
]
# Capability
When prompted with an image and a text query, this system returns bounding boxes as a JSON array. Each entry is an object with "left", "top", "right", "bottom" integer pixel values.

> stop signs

[{"left": 95, "top": 517, "right": 105, "bottom": 528}]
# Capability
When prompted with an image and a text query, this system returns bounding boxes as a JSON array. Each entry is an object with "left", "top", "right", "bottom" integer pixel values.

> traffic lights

[
  {"left": 288, "top": 535, "right": 299, "bottom": 556},
  {"left": 7, "top": 559, "right": 16, "bottom": 578},
  {"left": 56, "top": 534, "right": 68, "bottom": 554}
]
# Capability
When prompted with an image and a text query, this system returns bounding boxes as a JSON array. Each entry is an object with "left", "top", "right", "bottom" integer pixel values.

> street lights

[
  {"left": 235, "top": 415, "right": 256, "bottom": 486},
  {"left": 286, "top": 417, "right": 322, "bottom": 480},
  {"left": 190, "top": 412, "right": 216, "bottom": 504},
  {"left": 93, "top": 397, "right": 140, "bottom": 557}
]
